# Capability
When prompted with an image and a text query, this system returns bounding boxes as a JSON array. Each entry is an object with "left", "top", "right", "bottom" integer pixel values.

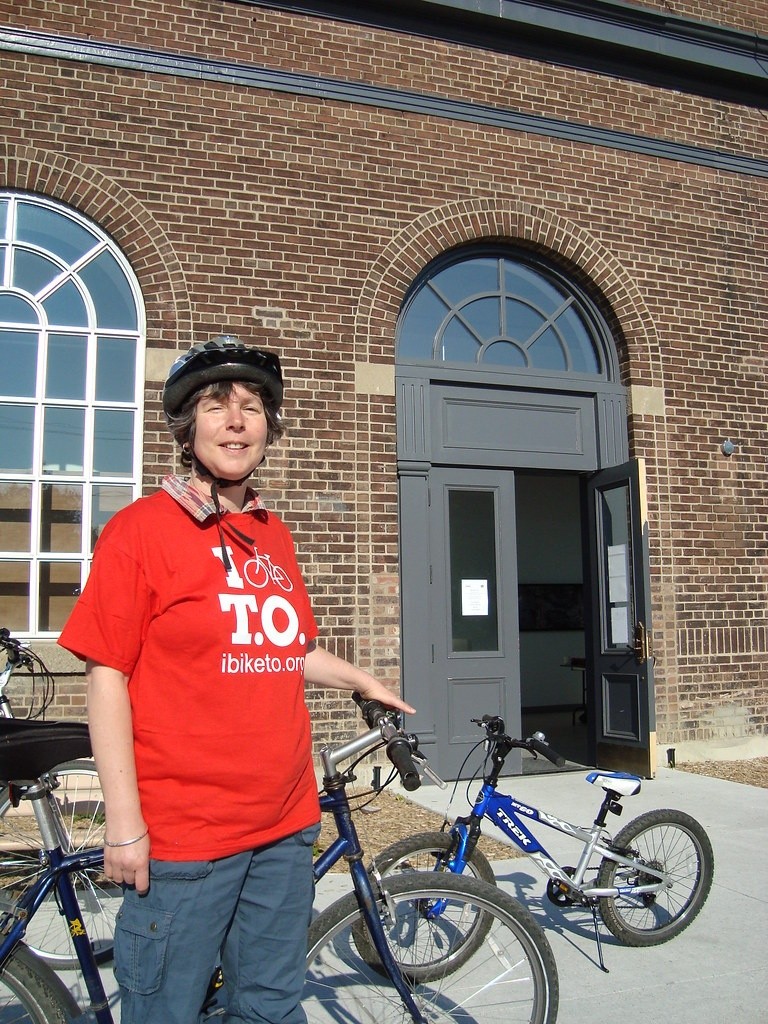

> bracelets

[{"left": 104, "top": 824, "right": 149, "bottom": 846}]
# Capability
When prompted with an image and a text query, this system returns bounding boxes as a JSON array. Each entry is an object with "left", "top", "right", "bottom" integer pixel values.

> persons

[{"left": 58, "top": 339, "right": 416, "bottom": 1024}]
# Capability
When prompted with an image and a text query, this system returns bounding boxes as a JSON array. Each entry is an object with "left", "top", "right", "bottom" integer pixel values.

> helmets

[{"left": 164, "top": 336, "right": 284, "bottom": 430}]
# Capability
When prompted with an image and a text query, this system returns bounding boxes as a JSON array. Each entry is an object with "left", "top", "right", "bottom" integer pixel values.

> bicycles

[
  {"left": 0, "top": 627, "right": 125, "bottom": 973},
  {"left": 0, "top": 692, "right": 561, "bottom": 1024},
  {"left": 351, "top": 713, "right": 715, "bottom": 985}
]
